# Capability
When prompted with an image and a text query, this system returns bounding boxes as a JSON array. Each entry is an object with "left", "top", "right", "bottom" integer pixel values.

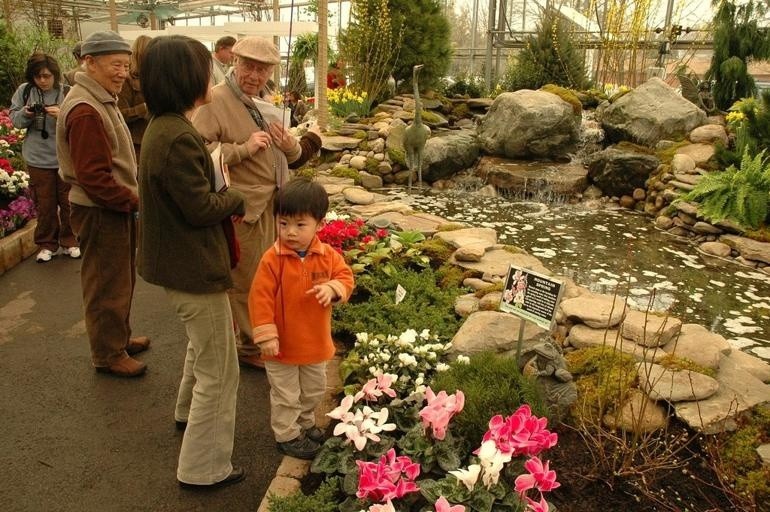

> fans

[
  {"left": 160, "top": 11, "right": 176, "bottom": 28},
  {"left": 135, "top": 11, "right": 150, "bottom": 28}
]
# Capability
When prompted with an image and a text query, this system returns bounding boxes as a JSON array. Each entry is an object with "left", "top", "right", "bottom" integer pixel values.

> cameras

[{"left": 27, "top": 101, "right": 50, "bottom": 117}]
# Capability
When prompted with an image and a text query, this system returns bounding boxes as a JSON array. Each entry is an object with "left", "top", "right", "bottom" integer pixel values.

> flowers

[{"left": 503, "top": 268, "right": 528, "bottom": 312}]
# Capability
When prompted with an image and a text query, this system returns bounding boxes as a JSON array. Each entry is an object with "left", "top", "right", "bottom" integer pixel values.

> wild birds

[{"left": 401, "top": 63, "right": 432, "bottom": 195}]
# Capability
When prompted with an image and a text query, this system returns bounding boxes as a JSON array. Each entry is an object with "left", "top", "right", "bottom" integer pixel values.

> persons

[
  {"left": 191, "top": 38, "right": 304, "bottom": 372},
  {"left": 247, "top": 178, "right": 357, "bottom": 459},
  {"left": 135, "top": 33, "right": 251, "bottom": 491},
  {"left": 325, "top": 56, "right": 350, "bottom": 89},
  {"left": 10, "top": 52, "right": 84, "bottom": 263},
  {"left": 56, "top": 30, "right": 149, "bottom": 379},
  {"left": 60, "top": 36, "right": 319, "bottom": 175}
]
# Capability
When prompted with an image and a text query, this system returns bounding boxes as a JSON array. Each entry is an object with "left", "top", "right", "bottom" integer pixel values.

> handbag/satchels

[{"left": 225, "top": 216, "right": 241, "bottom": 268}]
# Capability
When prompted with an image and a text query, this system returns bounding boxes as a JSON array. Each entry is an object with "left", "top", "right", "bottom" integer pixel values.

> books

[{"left": 209, "top": 142, "right": 231, "bottom": 193}]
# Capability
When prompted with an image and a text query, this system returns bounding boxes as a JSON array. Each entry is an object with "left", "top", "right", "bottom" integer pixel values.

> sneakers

[
  {"left": 276, "top": 435, "right": 321, "bottom": 460},
  {"left": 307, "top": 426, "right": 329, "bottom": 443},
  {"left": 64, "top": 246, "right": 81, "bottom": 258},
  {"left": 36, "top": 249, "right": 57, "bottom": 262}
]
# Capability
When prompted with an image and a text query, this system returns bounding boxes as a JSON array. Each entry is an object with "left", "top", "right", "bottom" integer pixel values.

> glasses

[{"left": 35, "top": 73, "right": 53, "bottom": 79}]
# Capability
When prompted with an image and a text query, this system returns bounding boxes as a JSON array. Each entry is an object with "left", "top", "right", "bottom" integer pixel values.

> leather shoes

[
  {"left": 178, "top": 461, "right": 247, "bottom": 490},
  {"left": 96, "top": 357, "right": 147, "bottom": 377},
  {"left": 127, "top": 336, "right": 150, "bottom": 355}
]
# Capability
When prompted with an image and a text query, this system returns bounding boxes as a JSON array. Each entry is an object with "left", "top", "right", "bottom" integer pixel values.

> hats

[
  {"left": 80, "top": 29, "right": 132, "bottom": 56},
  {"left": 231, "top": 36, "right": 281, "bottom": 65}
]
